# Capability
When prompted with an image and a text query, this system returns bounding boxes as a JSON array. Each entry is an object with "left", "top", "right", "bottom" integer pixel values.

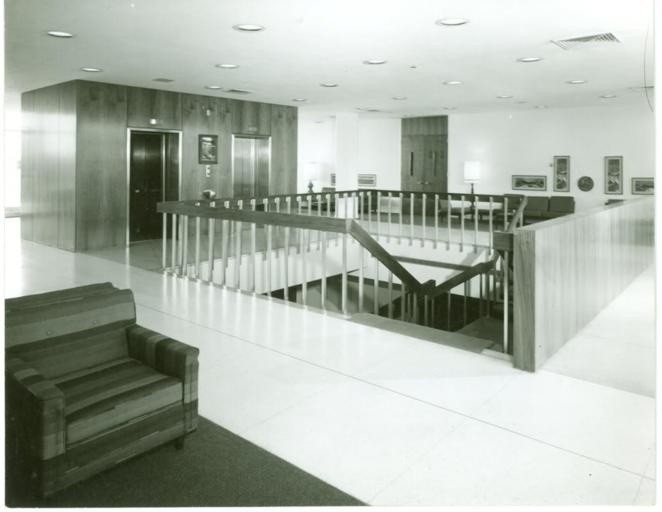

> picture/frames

[
  {"left": 554, "top": 155, "right": 571, "bottom": 192},
  {"left": 512, "top": 174, "right": 547, "bottom": 191},
  {"left": 577, "top": 176, "right": 594, "bottom": 191},
  {"left": 604, "top": 155, "right": 623, "bottom": 195},
  {"left": 198, "top": 133, "right": 218, "bottom": 164},
  {"left": 631, "top": 177, "right": 654, "bottom": 195}
]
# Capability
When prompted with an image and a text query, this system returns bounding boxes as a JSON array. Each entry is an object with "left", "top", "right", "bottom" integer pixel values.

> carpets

[{"left": 5, "top": 414, "right": 368, "bottom": 506}]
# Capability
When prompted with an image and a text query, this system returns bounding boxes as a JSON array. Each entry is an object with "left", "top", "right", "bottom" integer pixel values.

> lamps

[{"left": 463, "top": 160, "right": 481, "bottom": 206}]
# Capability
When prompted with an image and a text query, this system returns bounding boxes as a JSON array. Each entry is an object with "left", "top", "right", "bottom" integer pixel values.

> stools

[
  {"left": 471, "top": 208, "right": 500, "bottom": 224},
  {"left": 440, "top": 207, "right": 472, "bottom": 224},
  {"left": 495, "top": 212, "right": 515, "bottom": 224}
]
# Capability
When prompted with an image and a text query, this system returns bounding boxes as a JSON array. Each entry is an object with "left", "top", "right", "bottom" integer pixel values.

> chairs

[
  {"left": 543, "top": 195, "right": 576, "bottom": 219},
  {"left": 523, "top": 196, "right": 549, "bottom": 226},
  {"left": 496, "top": 195, "right": 526, "bottom": 230},
  {"left": 6, "top": 281, "right": 199, "bottom": 498}
]
